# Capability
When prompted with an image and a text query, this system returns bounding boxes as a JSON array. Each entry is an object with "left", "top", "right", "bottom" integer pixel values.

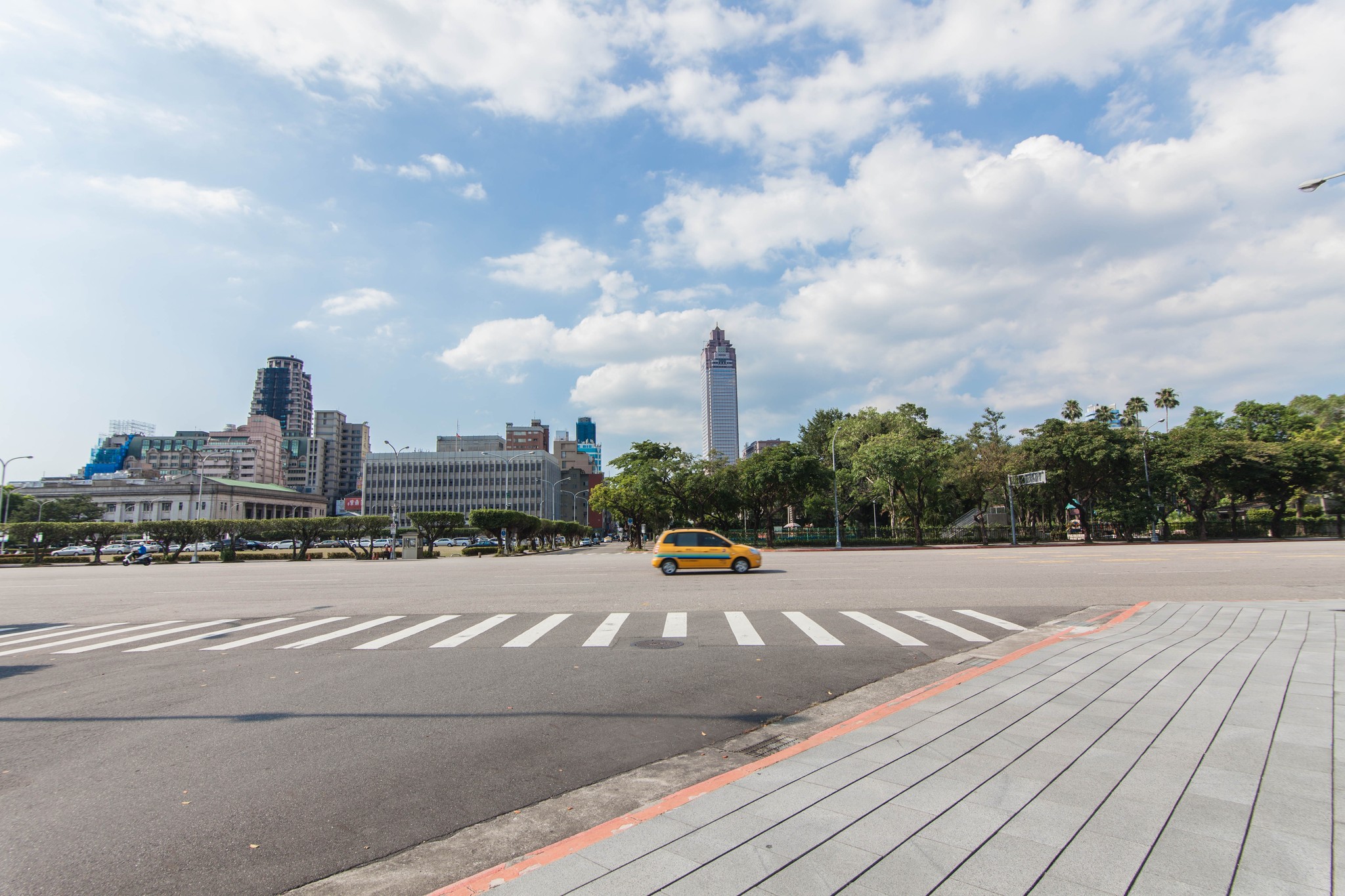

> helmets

[{"left": 139, "top": 542, "right": 145, "bottom": 545}]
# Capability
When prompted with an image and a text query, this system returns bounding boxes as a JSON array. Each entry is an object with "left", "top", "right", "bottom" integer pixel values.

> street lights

[
  {"left": 831, "top": 426, "right": 844, "bottom": 548},
  {"left": 113, "top": 504, "right": 137, "bottom": 523},
  {"left": 560, "top": 489, "right": 590, "bottom": 547},
  {"left": 25, "top": 499, "right": 57, "bottom": 522},
  {"left": 576, "top": 496, "right": 590, "bottom": 526},
  {"left": 0, "top": 456, "right": 34, "bottom": 523},
  {"left": 286, "top": 504, "right": 303, "bottom": 518},
  {"left": 1142, "top": 418, "right": 1165, "bottom": 545},
  {"left": 218, "top": 502, "right": 244, "bottom": 519},
  {"left": 384, "top": 440, "right": 410, "bottom": 560},
  {"left": 136, "top": 496, "right": 165, "bottom": 522},
  {"left": 181, "top": 444, "right": 226, "bottom": 564},
  {"left": 303, "top": 511, "right": 313, "bottom": 518},
  {"left": 535, "top": 477, "right": 572, "bottom": 521},
  {"left": 481, "top": 451, "right": 535, "bottom": 511},
  {"left": 371, "top": 503, "right": 379, "bottom": 516}
]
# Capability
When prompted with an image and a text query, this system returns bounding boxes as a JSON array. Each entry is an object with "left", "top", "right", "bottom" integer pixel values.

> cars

[
  {"left": 591, "top": 538, "right": 600, "bottom": 546},
  {"left": 601, "top": 533, "right": 635, "bottom": 543},
  {"left": 433, "top": 538, "right": 457, "bottom": 547},
  {"left": 651, "top": 526, "right": 762, "bottom": 575},
  {"left": 579, "top": 538, "right": 591, "bottom": 547},
  {"left": 423, "top": 539, "right": 429, "bottom": 546},
  {"left": 463, "top": 540, "right": 498, "bottom": 548},
  {"left": 452, "top": 536, "right": 565, "bottom": 546},
  {"left": 50, "top": 538, "right": 416, "bottom": 556}
]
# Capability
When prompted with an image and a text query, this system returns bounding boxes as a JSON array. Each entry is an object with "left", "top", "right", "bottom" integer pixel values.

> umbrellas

[{"left": 784, "top": 522, "right": 801, "bottom": 531}]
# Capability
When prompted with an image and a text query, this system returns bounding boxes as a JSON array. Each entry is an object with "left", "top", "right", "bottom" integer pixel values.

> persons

[
  {"left": 4, "top": 548, "right": 9, "bottom": 554},
  {"left": 18, "top": 547, "right": 24, "bottom": 553},
  {"left": 131, "top": 542, "right": 146, "bottom": 560},
  {"left": 656, "top": 534, "right": 659, "bottom": 542},
  {"left": 641, "top": 535, "right": 644, "bottom": 547}
]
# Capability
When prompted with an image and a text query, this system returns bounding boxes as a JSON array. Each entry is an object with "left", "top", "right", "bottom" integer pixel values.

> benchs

[
  {"left": 1171, "top": 529, "right": 1187, "bottom": 536},
  {"left": 1145, "top": 530, "right": 1160, "bottom": 534}
]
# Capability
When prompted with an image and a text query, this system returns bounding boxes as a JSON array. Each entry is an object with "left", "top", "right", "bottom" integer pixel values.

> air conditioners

[
  {"left": 179, "top": 504, "right": 182, "bottom": 506},
  {"left": 146, "top": 504, "right": 149, "bottom": 506},
  {"left": 233, "top": 460, "right": 236, "bottom": 463},
  {"left": 109, "top": 505, "right": 113, "bottom": 507},
  {"left": 202, "top": 503, "right": 205, "bottom": 506},
  {"left": 225, "top": 459, "right": 229, "bottom": 462},
  {"left": 235, "top": 475, "right": 238, "bottom": 478},
  {"left": 197, "top": 459, "right": 202, "bottom": 461},
  {"left": 214, "top": 459, "right": 217, "bottom": 461},
  {"left": 164, "top": 504, "right": 168, "bottom": 506},
  {"left": 128, "top": 505, "right": 131, "bottom": 507},
  {"left": 235, "top": 468, "right": 238, "bottom": 470}
]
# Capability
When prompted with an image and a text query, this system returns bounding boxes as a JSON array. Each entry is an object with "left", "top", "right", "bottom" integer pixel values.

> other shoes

[{"left": 131, "top": 559, "right": 135, "bottom": 561}]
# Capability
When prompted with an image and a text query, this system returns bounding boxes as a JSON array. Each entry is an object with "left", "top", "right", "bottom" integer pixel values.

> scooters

[{"left": 122, "top": 551, "right": 153, "bottom": 566}]
